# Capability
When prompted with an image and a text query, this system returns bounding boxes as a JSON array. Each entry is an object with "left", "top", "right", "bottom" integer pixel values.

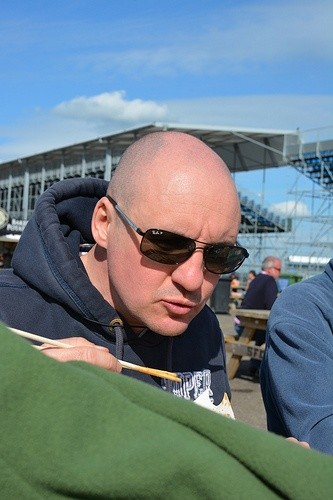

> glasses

[
  {"left": 273, "top": 267, "right": 281, "bottom": 272},
  {"left": 106, "top": 195, "right": 249, "bottom": 274}
]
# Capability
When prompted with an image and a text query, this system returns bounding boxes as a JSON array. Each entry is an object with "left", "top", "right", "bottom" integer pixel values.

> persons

[
  {"left": 263, "top": 259, "right": 333, "bottom": 456},
  {"left": 0, "top": 130, "right": 310, "bottom": 451},
  {"left": 242, "top": 256, "right": 281, "bottom": 377},
  {"left": 246, "top": 270, "right": 256, "bottom": 289},
  {"left": 230, "top": 271, "right": 239, "bottom": 308}
]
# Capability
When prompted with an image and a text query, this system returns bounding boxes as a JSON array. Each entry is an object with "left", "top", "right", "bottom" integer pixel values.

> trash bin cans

[{"left": 211, "top": 277, "right": 232, "bottom": 313}]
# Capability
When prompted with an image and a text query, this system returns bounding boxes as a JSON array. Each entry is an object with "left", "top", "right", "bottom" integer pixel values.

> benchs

[{"left": 224, "top": 335, "right": 251, "bottom": 380}]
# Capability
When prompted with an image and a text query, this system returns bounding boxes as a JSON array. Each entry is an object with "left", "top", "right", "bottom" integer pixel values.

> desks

[{"left": 227, "top": 308, "right": 271, "bottom": 380}]
